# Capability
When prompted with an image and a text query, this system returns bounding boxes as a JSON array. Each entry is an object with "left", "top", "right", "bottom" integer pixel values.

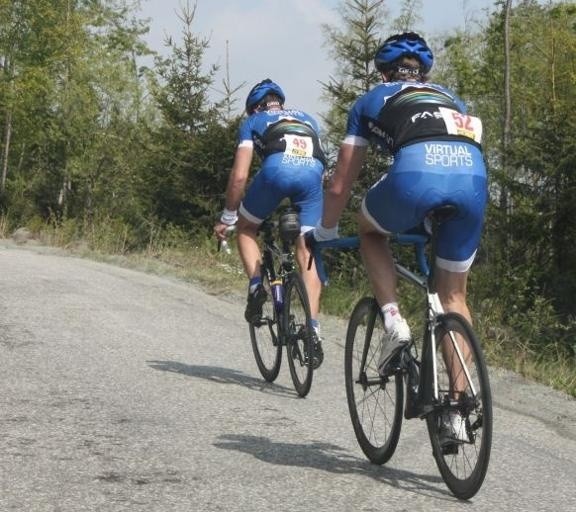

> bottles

[{"left": 271, "top": 280, "right": 286, "bottom": 307}]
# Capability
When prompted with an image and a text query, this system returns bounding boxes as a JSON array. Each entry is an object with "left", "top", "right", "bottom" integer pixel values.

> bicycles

[
  {"left": 213, "top": 202, "right": 315, "bottom": 401},
  {"left": 300, "top": 198, "right": 505, "bottom": 502}
]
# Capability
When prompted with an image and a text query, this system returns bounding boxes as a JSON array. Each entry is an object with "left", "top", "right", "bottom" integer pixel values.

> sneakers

[
  {"left": 243, "top": 284, "right": 268, "bottom": 323},
  {"left": 302, "top": 325, "right": 326, "bottom": 370},
  {"left": 431, "top": 397, "right": 473, "bottom": 446},
  {"left": 374, "top": 317, "right": 413, "bottom": 375}
]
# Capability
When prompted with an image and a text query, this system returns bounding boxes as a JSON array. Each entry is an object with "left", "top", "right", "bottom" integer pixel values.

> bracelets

[
  {"left": 313, "top": 216, "right": 340, "bottom": 242},
  {"left": 219, "top": 207, "right": 239, "bottom": 226}
]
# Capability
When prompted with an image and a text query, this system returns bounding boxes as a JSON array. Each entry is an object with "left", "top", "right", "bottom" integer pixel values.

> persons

[
  {"left": 214, "top": 77, "right": 336, "bottom": 370},
  {"left": 317, "top": 30, "right": 492, "bottom": 447}
]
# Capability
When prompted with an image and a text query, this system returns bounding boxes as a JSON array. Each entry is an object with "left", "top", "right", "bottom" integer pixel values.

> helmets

[
  {"left": 244, "top": 78, "right": 289, "bottom": 115},
  {"left": 372, "top": 29, "right": 434, "bottom": 76}
]
